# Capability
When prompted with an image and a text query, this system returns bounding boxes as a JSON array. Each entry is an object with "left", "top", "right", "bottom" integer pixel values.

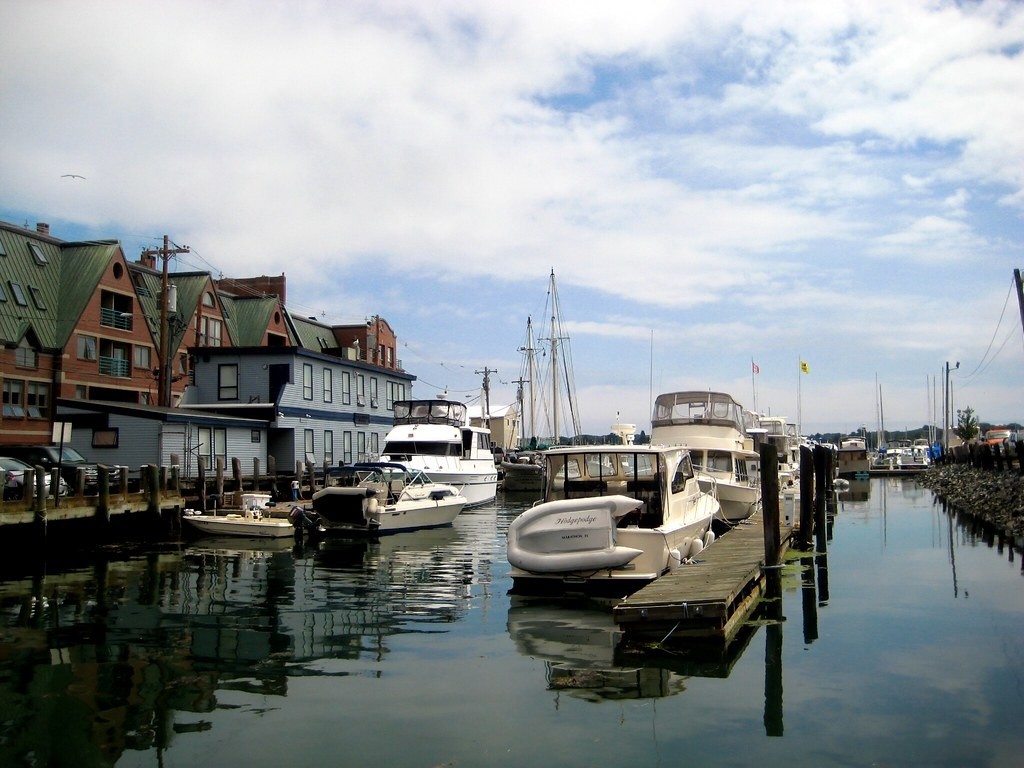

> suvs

[{"left": 0, "top": 440, "right": 120, "bottom": 497}]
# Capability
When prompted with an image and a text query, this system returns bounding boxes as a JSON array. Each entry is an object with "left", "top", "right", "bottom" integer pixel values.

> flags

[
  {"left": 799, "top": 360, "right": 809, "bottom": 374},
  {"left": 752, "top": 362, "right": 759, "bottom": 374}
]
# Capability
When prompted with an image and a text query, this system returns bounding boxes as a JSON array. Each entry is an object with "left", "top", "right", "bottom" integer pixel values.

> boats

[
  {"left": 745, "top": 351, "right": 983, "bottom": 524},
  {"left": 650, "top": 390, "right": 772, "bottom": 522},
  {"left": 506, "top": 440, "right": 729, "bottom": 579},
  {"left": 209, "top": 461, "right": 468, "bottom": 529},
  {"left": 185, "top": 485, "right": 382, "bottom": 541},
  {"left": 374, "top": 397, "right": 497, "bottom": 506}
]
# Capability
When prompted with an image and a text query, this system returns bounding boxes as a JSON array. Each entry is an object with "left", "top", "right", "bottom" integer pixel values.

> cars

[
  {"left": 986, "top": 429, "right": 1012, "bottom": 448},
  {"left": 0, "top": 456, "right": 68, "bottom": 501}
]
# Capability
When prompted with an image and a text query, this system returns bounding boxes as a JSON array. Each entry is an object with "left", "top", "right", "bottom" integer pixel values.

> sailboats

[{"left": 495, "top": 263, "right": 656, "bottom": 496}]
